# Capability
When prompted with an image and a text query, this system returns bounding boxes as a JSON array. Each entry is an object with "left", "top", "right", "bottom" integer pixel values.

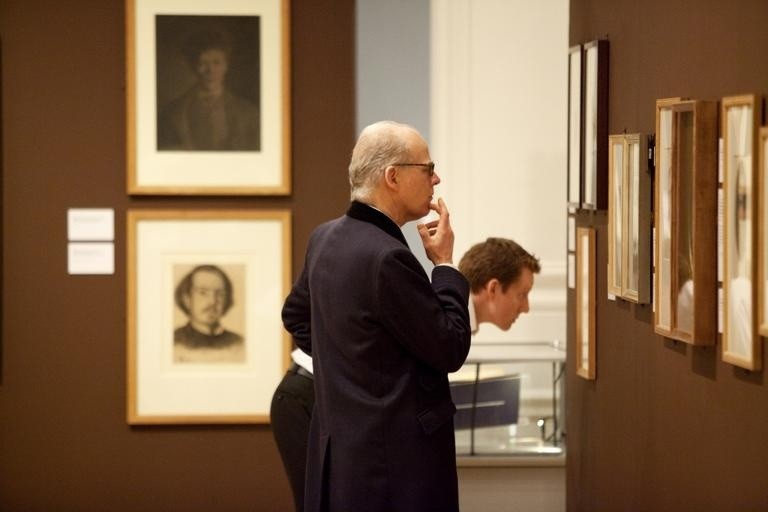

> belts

[{"left": 288, "top": 363, "right": 315, "bottom": 380}]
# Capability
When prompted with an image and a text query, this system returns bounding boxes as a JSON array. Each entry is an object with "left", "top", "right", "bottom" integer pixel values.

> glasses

[{"left": 394, "top": 162, "right": 435, "bottom": 176}]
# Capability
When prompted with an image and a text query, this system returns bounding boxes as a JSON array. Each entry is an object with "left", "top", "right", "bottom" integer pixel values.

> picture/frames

[
  {"left": 125, "top": 207, "right": 295, "bottom": 427},
  {"left": 124, "top": 1, "right": 293, "bottom": 197},
  {"left": 566, "top": 37, "right": 768, "bottom": 381}
]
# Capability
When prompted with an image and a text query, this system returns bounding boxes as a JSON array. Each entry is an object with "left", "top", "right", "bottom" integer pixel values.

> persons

[
  {"left": 169, "top": 264, "right": 246, "bottom": 351},
  {"left": 265, "top": 232, "right": 545, "bottom": 511},
  {"left": 155, "top": 32, "right": 263, "bottom": 154},
  {"left": 280, "top": 116, "right": 473, "bottom": 512}
]
